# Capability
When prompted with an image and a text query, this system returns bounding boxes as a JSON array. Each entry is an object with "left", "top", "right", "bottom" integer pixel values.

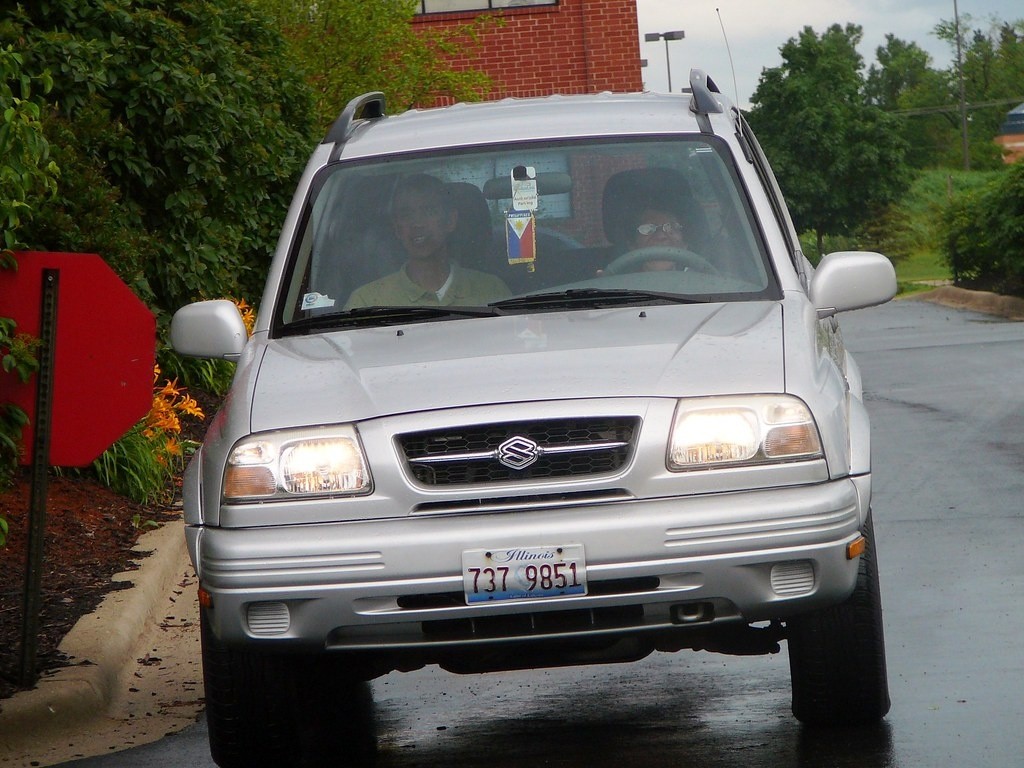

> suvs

[{"left": 169, "top": 69, "right": 898, "bottom": 768}]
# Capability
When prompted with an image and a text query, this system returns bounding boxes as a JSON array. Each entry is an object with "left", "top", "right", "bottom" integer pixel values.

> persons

[
  {"left": 587, "top": 192, "right": 699, "bottom": 273},
  {"left": 343, "top": 174, "right": 515, "bottom": 307}
]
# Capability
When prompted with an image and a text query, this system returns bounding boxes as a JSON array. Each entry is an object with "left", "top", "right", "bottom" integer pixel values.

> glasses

[{"left": 635, "top": 222, "right": 686, "bottom": 236}]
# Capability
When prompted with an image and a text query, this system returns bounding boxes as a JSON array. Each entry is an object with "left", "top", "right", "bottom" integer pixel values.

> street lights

[{"left": 644, "top": 30, "right": 686, "bottom": 93}]
